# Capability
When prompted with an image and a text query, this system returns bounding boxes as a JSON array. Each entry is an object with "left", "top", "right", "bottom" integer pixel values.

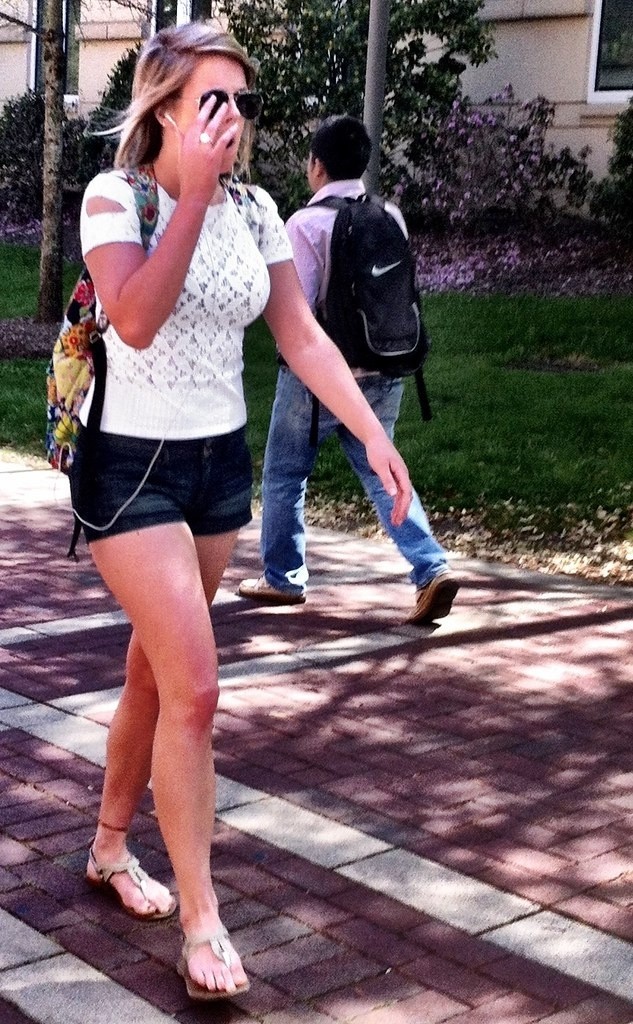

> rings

[{"left": 199, "top": 133, "right": 214, "bottom": 143}]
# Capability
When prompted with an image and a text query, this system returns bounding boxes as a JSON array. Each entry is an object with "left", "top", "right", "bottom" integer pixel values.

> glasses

[{"left": 170, "top": 89, "right": 264, "bottom": 120}]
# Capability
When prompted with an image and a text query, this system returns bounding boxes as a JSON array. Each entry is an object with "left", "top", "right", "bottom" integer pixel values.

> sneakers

[
  {"left": 239, "top": 572, "right": 306, "bottom": 604},
  {"left": 407, "top": 573, "right": 461, "bottom": 622}
]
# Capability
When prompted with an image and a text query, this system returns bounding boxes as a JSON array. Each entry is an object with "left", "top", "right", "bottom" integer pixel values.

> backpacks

[
  {"left": 45, "top": 160, "right": 263, "bottom": 474},
  {"left": 303, "top": 190, "right": 429, "bottom": 378}
]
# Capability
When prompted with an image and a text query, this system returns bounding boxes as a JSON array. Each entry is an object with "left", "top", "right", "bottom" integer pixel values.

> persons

[
  {"left": 234, "top": 114, "right": 461, "bottom": 624},
  {"left": 62, "top": 24, "right": 414, "bottom": 1001}
]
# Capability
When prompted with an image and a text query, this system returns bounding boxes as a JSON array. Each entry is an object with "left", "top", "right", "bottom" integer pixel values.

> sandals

[
  {"left": 86, "top": 845, "right": 177, "bottom": 922},
  {"left": 176, "top": 927, "right": 250, "bottom": 1000}
]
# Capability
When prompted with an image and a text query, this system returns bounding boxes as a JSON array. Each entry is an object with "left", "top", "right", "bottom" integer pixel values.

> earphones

[{"left": 163, "top": 110, "right": 176, "bottom": 127}]
